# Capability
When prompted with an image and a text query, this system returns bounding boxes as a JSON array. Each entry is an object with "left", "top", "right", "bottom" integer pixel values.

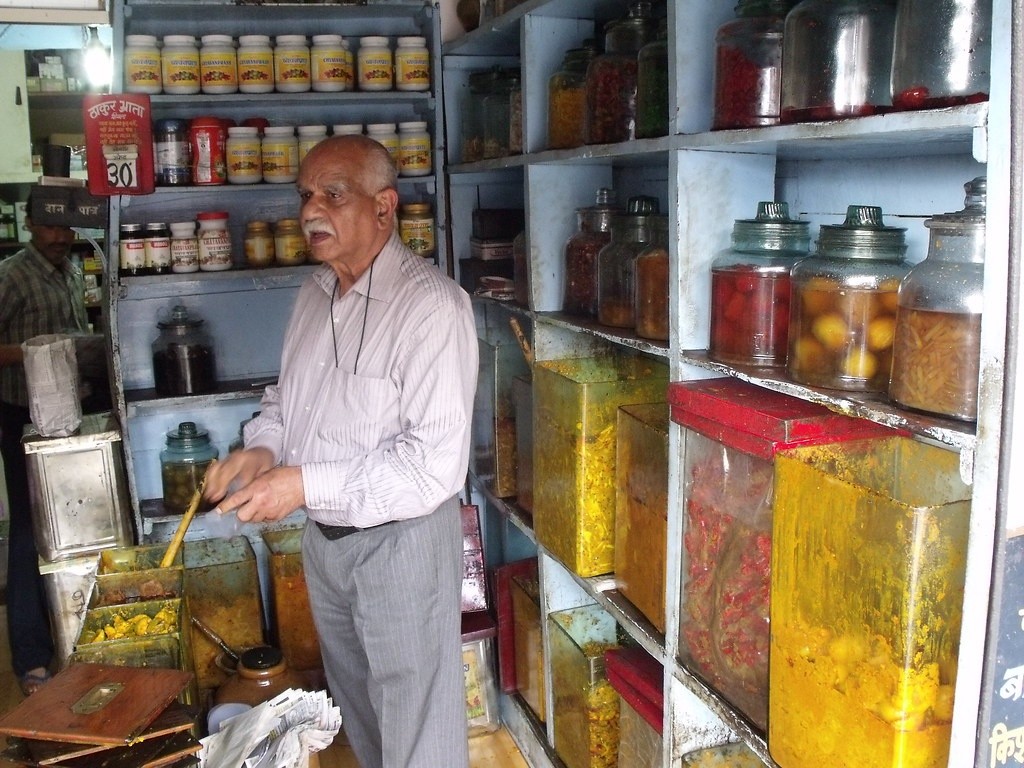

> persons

[
  {"left": 1, "top": 187, "right": 93, "bottom": 697},
  {"left": 202, "top": 134, "right": 481, "bottom": 768}
]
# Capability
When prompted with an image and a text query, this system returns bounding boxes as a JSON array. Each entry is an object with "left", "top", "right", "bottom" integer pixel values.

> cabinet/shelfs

[
  {"left": 0, "top": 50, "right": 39, "bottom": 184},
  {"left": 444, "top": 2, "right": 1014, "bottom": 768},
  {"left": 0, "top": 91, "right": 103, "bottom": 308},
  {"left": 109, "top": 0, "right": 446, "bottom": 634}
]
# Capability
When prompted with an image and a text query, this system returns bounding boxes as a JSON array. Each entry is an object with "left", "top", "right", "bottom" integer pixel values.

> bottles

[{"left": 216, "top": 646, "right": 320, "bottom": 768}]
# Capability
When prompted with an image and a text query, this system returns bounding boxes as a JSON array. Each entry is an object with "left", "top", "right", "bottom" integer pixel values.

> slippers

[{"left": 19, "top": 668, "right": 52, "bottom": 695}]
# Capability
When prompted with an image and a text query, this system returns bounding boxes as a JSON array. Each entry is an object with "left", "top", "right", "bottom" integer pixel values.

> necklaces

[{"left": 331, "top": 252, "right": 376, "bottom": 375}]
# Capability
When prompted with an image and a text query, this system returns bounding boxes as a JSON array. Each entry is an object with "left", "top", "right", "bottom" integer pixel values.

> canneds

[
  {"left": 245, "top": 220, "right": 274, "bottom": 267},
  {"left": 120, "top": 224, "right": 145, "bottom": 276},
  {"left": 400, "top": 203, "right": 435, "bottom": 257},
  {"left": 274, "top": 218, "right": 306, "bottom": 265},
  {"left": 124, "top": 35, "right": 429, "bottom": 91},
  {"left": 227, "top": 122, "right": 432, "bottom": 183},
  {"left": 144, "top": 223, "right": 171, "bottom": 275}
]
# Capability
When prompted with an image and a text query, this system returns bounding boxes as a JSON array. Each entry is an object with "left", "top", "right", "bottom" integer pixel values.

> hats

[{"left": 25, "top": 187, "right": 81, "bottom": 227}]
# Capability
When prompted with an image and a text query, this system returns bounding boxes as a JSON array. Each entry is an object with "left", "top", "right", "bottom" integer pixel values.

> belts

[{"left": 315, "top": 521, "right": 400, "bottom": 540}]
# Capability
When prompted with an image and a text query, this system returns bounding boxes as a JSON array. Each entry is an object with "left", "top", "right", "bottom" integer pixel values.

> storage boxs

[
  {"left": 681, "top": 742, "right": 770, "bottom": 768},
  {"left": 264, "top": 528, "right": 326, "bottom": 669},
  {"left": 604, "top": 647, "right": 664, "bottom": 768},
  {"left": 513, "top": 374, "right": 533, "bottom": 514},
  {"left": 532, "top": 354, "right": 670, "bottom": 577},
  {"left": 547, "top": 603, "right": 640, "bottom": 768},
  {"left": 770, "top": 438, "right": 973, "bottom": 768},
  {"left": 616, "top": 403, "right": 669, "bottom": 633},
  {"left": 472, "top": 326, "right": 531, "bottom": 499},
  {"left": 63, "top": 535, "right": 267, "bottom": 768},
  {"left": 667, "top": 378, "right": 913, "bottom": 735},
  {"left": 510, "top": 572, "right": 545, "bottom": 723},
  {"left": 459, "top": 612, "right": 499, "bottom": 739}
]
